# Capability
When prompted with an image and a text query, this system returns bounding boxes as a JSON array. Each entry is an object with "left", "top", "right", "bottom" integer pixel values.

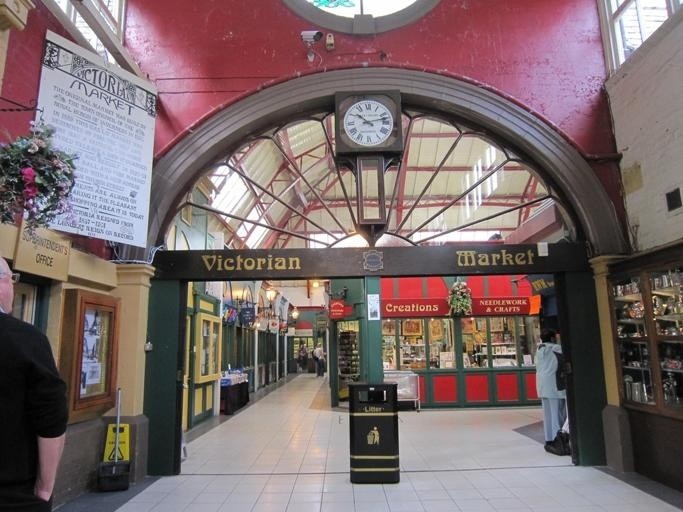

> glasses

[{"left": 10, "top": 273, "right": 20, "bottom": 283}]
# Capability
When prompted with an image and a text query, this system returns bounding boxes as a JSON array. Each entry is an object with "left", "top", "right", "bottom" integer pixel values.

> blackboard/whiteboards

[{"left": 383, "top": 371, "right": 420, "bottom": 401}]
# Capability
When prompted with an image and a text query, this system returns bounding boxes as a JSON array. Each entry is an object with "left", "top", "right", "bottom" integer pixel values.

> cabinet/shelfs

[{"left": 605, "top": 239, "right": 683, "bottom": 490}]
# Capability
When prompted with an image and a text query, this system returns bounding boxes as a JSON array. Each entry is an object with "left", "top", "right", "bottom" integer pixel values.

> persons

[
  {"left": 0, "top": 255, "right": 71, "bottom": 512},
  {"left": 534, "top": 326, "right": 569, "bottom": 441},
  {"left": 298, "top": 340, "right": 308, "bottom": 371}
]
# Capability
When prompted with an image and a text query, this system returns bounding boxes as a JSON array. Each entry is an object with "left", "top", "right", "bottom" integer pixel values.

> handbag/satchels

[{"left": 545, "top": 432, "right": 570, "bottom": 455}]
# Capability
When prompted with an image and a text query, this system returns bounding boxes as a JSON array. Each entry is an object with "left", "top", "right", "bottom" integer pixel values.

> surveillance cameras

[{"left": 301, "top": 30, "right": 322, "bottom": 46}]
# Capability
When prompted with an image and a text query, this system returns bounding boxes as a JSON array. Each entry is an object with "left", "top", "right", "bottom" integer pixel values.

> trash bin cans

[{"left": 348, "top": 382, "right": 400, "bottom": 484}]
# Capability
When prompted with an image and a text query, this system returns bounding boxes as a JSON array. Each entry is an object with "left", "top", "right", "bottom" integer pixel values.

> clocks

[{"left": 334, "top": 90, "right": 403, "bottom": 227}]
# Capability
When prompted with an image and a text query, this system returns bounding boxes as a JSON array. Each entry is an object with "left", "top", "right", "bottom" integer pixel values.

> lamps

[
  {"left": 291, "top": 306, "right": 298, "bottom": 319},
  {"left": 265, "top": 286, "right": 276, "bottom": 304}
]
